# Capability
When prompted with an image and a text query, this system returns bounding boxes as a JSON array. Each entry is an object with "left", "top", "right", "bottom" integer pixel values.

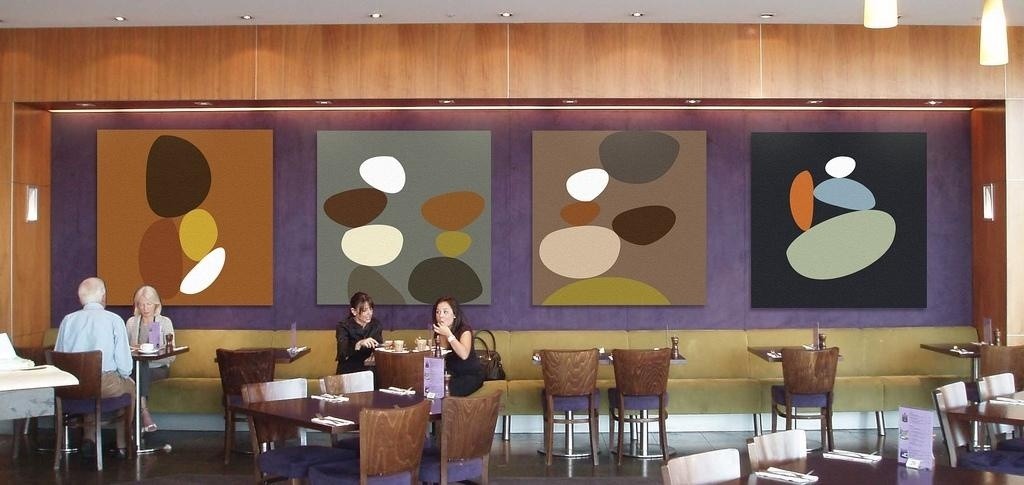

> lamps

[
  {"left": 978, "top": 0, "right": 1010, "bottom": 67},
  {"left": 863, "top": 0, "right": 899, "bottom": 30}
]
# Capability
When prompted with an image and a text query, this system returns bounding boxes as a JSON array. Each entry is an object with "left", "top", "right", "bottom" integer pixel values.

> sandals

[{"left": 145, "top": 424, "right": 158, "bottom": 432}]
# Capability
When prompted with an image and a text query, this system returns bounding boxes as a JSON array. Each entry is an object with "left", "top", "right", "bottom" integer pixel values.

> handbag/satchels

[{"left": 473, "top": 330, "right": 505, "bottom": 380}]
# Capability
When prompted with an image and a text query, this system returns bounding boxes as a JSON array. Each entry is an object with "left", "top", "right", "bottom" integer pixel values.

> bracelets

[{"left": 447, "top": 335, "right": 457, "bottom": 345}]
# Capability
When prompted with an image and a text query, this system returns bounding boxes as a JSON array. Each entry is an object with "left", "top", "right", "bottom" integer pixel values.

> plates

[{"left": 138, "top": 350, "right": 158, "bottom": 353}]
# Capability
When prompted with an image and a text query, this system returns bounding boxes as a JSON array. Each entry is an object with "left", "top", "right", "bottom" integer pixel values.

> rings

[{"left": 369, "top": 341, "right": 372, "bottom": 343}]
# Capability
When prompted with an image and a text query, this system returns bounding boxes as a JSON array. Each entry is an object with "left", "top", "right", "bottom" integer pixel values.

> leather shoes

[{"left": 107, "top": 448, "right": 128, "bottom": 454}]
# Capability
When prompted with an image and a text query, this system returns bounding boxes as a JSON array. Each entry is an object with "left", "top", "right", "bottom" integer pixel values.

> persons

[
  {"left": 429, "top": 296, "right": 486, "bottom": 399},
  {"left": 334, "top": 289, "right": 383, "bottom": 390},
  {"left": 124, "top": 285, "right": 175, "bottom": 440},
  {"left": 50, "top": 276, "right": 138, "bottom": 460}
]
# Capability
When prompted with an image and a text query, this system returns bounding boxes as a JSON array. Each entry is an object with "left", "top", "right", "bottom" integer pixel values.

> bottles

[
  {"left": 433, "top": 334, "right": 442, "bottom": 359},
  {"left": 819, "top": 334, "right": 827, "bottom": 350},
  {"left": 165, "top": 332, "right": 173, "bottom": 354},
  {"left": 671, "top": 336, "right": 679, "bottom": 359},
  {"left": 993, "top": 329, "right": 1000, "bottom": 347},
  {"left": 443, "top": 370, "right": 453, "bottom": 397}
]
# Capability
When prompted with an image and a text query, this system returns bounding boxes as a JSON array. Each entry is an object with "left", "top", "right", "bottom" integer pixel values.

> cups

[
  {"left": 139, "top": 343, "right": 153, "bottom": 351},
  {"left": 384, "top": 339, "right": 426, "bottom": 353}
]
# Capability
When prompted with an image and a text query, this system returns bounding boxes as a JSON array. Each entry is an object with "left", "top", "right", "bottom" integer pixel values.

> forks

[
  {"left": 824, "top": 447, "right": 881, "bottom": 461},
  {"left": 757, "top": 466, "right": 816, "bottom": 481}
]
